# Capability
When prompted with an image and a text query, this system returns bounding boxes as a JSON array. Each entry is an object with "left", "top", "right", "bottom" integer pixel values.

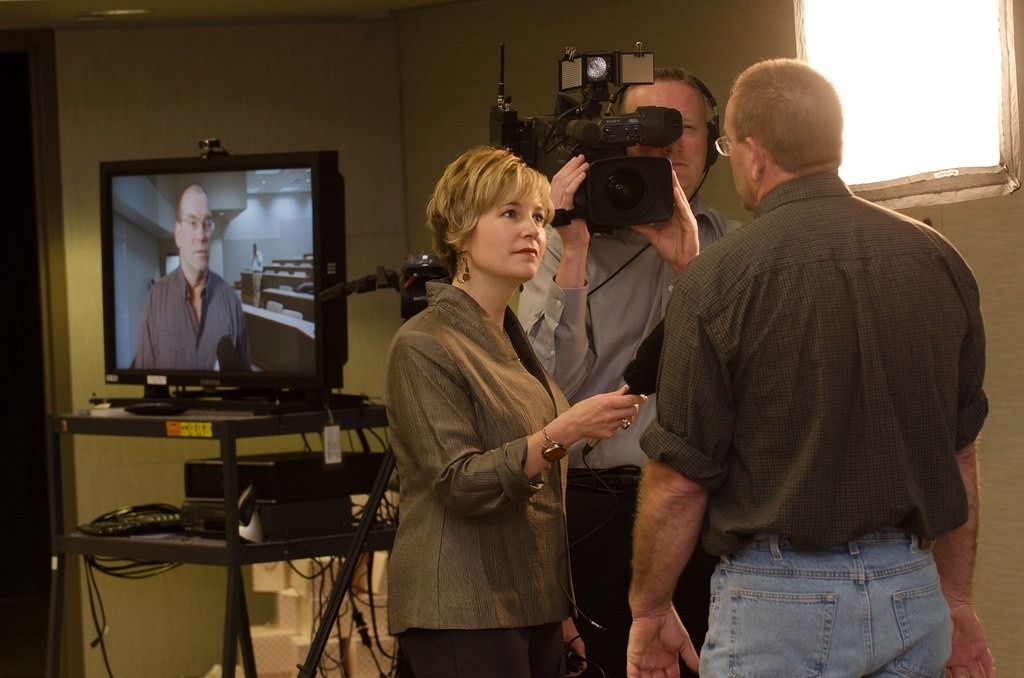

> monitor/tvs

[{"left": 99, "top": 150, "right": 363, "bottom": 415}]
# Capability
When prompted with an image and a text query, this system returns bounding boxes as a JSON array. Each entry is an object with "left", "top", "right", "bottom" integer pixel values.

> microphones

[
  {"left": 581, "top": 317, "right": 667, "bottom": 455},
  {"left": 566, "top": 120, "right": 602, "bottom": 146}
]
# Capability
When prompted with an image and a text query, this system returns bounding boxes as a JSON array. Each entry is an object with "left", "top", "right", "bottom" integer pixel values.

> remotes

[{"left": 125, "top": 397, "right": 190, "bottom": 415}]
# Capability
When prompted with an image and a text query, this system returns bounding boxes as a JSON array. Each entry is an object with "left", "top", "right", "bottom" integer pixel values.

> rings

[{"left": 621, "top": 419, "right": 631, "bottom": 429}]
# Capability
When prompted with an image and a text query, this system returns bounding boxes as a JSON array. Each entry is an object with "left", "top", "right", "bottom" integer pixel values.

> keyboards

[{"left": 78, "top": 512, "right": 180, "bottom": 536}]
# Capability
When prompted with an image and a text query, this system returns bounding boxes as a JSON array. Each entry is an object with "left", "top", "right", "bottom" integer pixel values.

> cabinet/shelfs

[{"left": 41, "top": 402, "right": 412, "bottom": 678}]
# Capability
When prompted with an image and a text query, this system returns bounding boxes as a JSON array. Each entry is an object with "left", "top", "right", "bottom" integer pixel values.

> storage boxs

[{"left": 205, "top": 551, "right": 399, "bottom": 678}]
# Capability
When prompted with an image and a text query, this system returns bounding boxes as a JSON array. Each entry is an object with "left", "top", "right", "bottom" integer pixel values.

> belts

[{"left": 565, "top": 470, "right": 641, "bottom": 492}]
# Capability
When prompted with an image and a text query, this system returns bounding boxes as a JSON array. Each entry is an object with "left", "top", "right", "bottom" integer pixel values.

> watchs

[{"left": 541, "top": 427, "right": 568, "bottom": 463}]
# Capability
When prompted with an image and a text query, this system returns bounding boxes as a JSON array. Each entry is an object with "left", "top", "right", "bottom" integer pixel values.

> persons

[
  {"left": 520, "top": 67, "right": 747, "bottom": 678},
  {"left": 387, "top": 144, "right": 650, "bottom": 678},
  {"left": 134, "top": 181, "right": 250, "bottom": 372},
  {"left": 251, "top": 243, "right": 263, "bottom": 272},
  {"left": 625, "top": 60, "right": 1000, "bottom": 678}
]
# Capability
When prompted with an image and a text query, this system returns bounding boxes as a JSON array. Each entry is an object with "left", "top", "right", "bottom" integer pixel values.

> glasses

[
  {"left": 714, "top": 135, "right": 763, "bottom": 156},
  {"left": 178, "top": 218, "right": 215, "bottom": 233}
]
